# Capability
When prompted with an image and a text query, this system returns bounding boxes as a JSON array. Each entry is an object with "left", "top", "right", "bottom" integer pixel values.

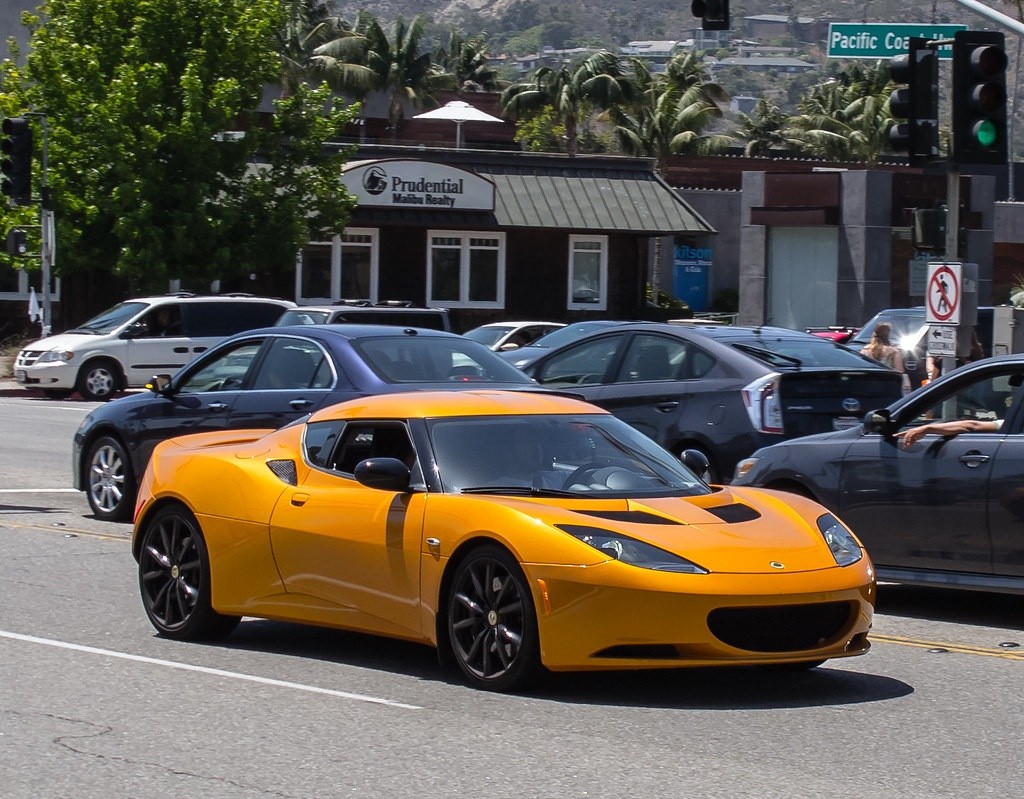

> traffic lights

[
  {"left": 0, "top": 115, "right": 32, "bottom": 209},
  {"left": 885, "top": 37, "right": 942, "bottom": 165},
  {"left": 951, "top": 30, "right": 1009, "bottom": 173}
]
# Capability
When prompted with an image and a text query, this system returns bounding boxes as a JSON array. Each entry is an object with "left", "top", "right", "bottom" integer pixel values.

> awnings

[{"left": 468, "top": 162, "right": 719, "bottom": 232}]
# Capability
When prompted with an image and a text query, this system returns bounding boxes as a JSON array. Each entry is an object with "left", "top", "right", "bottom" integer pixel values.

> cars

[
  {"left": 520, "top": 321, "right": 906, "bottom": 488},
  {"left": 460, "top": 320, "right": 568, "bottom": 354},
  {"left": 729, "top": 352, "right": 1023, "bottom": 595},
  {"left": 71, "top": 324, "right": 586, "bottom": 524}
]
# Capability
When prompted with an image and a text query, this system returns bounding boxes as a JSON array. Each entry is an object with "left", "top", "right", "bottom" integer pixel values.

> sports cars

[{"left": 131, "top": 389, "right": 877, "bottom": 694}]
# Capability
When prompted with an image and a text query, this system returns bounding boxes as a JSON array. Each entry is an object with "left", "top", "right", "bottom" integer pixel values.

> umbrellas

[{"left": 410, "top": 100, "right": 507, "bottom": 147}]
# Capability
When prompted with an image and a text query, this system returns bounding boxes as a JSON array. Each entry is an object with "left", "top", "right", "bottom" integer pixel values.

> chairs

[
  {"left": 637, "top": 344, "right": 671, "bottom": 378},
  {"left": 369, "top": 429, "right": 415, "bottom": 468},
  {"left": 267, "top": 349, "right": 319, "bottom": 390}
]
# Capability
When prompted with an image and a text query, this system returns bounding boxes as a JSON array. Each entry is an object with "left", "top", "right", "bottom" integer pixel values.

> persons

[
  {"left": 858, "top": 323, "right": 903, "bottom": 373},
  {"left": 891, "top": 418, "right": 1005, "bottom": 448},
  {"left": 925, "top": 329, "right": 985, "bottom": 419}
]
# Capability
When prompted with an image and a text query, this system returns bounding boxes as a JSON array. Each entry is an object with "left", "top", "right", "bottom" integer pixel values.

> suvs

[{"left": 213, "top": 304, "right": 450, "bottom": 379}]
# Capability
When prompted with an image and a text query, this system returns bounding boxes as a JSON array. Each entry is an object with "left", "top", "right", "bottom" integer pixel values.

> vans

[
  {"left": 846, "top": 304, "right": 995, "bottom": 391},
  {"left": 12, "top": 291, "right": 316, "bottom": 401}
]
